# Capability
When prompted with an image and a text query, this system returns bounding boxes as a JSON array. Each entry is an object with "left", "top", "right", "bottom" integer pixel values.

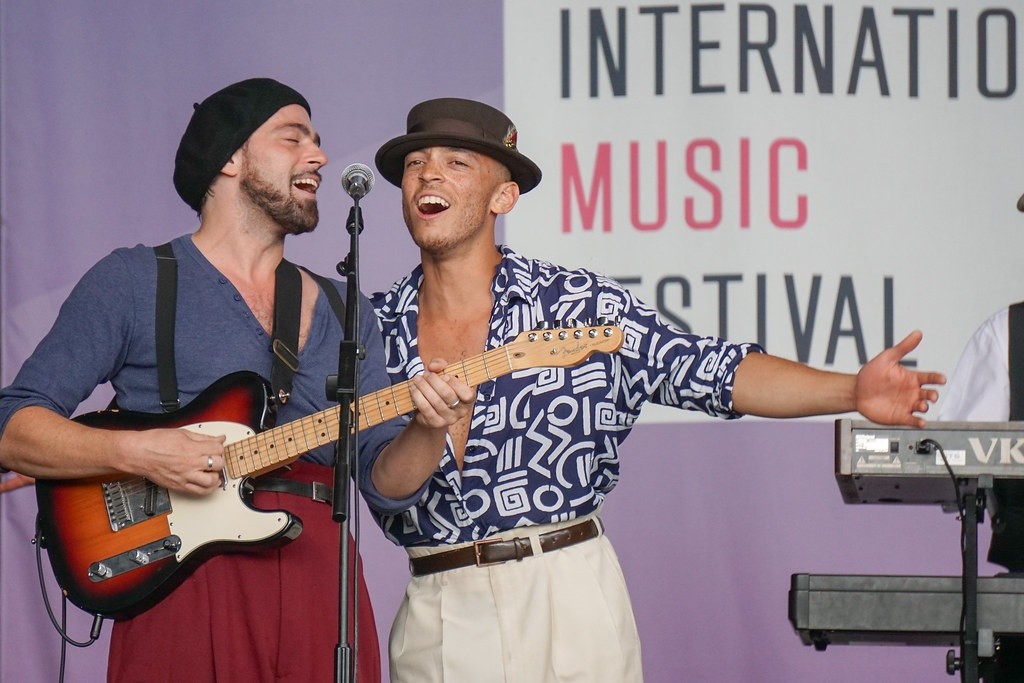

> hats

[
  {"left": 172, "top": 76, "right": 311, "bottom": 209},
  {"left": 372, "top": 97, "right": 543, "bottom": 193}
]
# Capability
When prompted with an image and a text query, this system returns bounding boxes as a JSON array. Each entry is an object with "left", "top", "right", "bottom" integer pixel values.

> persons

[
  {"left": 939, "top": 290, "right": 1024, "bottom": 572},
  {"left": 353, "top": 97, "right": 948, "bottom": 683},
  {"left": 0, "top": 74, "right": 478, "bottom": 683}
]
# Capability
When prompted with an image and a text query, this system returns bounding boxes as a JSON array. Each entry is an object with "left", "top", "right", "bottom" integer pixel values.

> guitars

[{"left": 36, "top": 322, "right": 625, "bottom": 620}]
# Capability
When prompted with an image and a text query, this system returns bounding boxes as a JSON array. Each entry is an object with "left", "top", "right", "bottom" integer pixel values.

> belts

[
  {"left": 249, "top": 476, "right": 333, "bottom": 504},
  {"left": 410, "top": 516, "right": 606, "bottom": 575}
]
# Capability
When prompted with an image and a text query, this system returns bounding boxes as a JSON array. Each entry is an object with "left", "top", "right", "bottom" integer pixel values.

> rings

[
  {"left": 205, "top": 456, "right": 215, "bottom": 471},
  {"left": 446, "top": 399, "right": 460, "bottom": 410}
]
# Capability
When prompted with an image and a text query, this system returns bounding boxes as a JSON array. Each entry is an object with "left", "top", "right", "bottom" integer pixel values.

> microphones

[{"left": 341, "top": 163, "right": 375, "bottom": 200}]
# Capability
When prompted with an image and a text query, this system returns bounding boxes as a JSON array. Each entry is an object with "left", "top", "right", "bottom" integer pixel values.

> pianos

[
  {"left": 788, "top": 573, "right": 1022, "bottom": 652},
  {"left": 836, "top": 415, "right": 1024, "bottom": 683}
]
